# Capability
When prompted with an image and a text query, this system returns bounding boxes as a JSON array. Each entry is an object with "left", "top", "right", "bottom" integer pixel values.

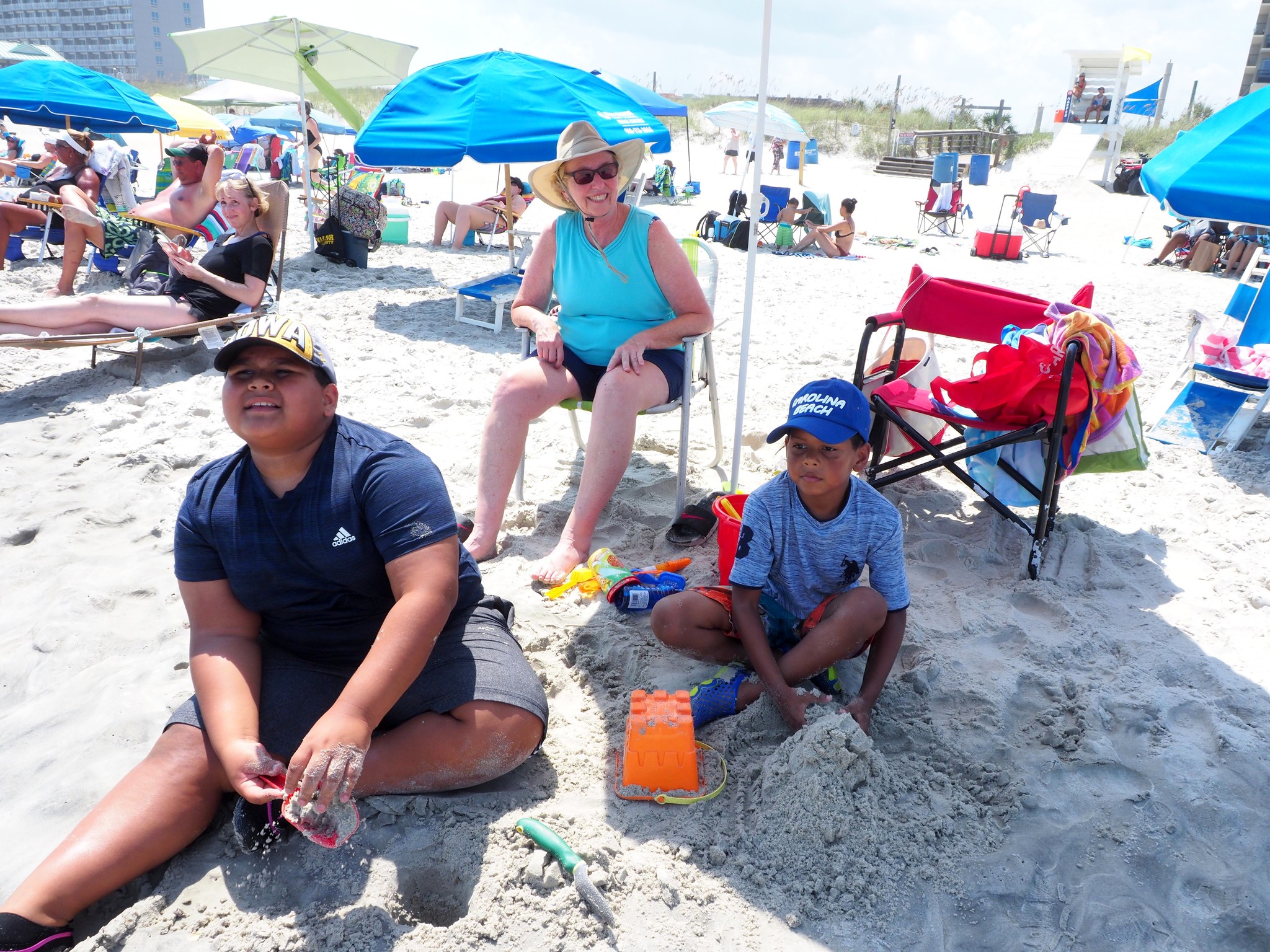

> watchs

[{"left": 54, "top": 194, "right": 58, "bottom": 204}]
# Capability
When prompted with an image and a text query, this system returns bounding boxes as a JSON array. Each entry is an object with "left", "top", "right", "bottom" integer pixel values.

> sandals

[{"left": 690, "top": 662, "right": 841, "bottom": 728}]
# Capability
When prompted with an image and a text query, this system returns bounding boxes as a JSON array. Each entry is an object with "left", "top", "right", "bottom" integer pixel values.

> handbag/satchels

[
  {"left": 1188, "top": 238, "right": 1224, "bottom": 273},
  {"left": 313, "top": 184, "right": 387, "bottom": 259},
  {"left": 387, "top": 178, "right": 405, "bottom": 196},
  {"left": 860, "top": 271, "right": 1090, "bottom": 457},
  {"left": 779, "top": 151, "right": 784, "bottom": 159}
]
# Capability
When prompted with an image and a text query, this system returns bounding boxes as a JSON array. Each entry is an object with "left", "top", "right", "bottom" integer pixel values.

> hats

[
  {"left": 5, "top": 134, "right": 20, "bottom": 144},
  {"left": 42, "top": 135, "right": 57, "bottom": 144},
  {"left": 504, "top": 175, "right": 524, "bottom": 190},
  {"left": 528, "top": 121, "right": 645, "bottom": 212},
  {"left": 214, "top": 313, "right": 338, "bottom": 386},
  {"left": 48, "top": 129, "right": 90, "bottom": 157},
  {"left": 1023, "top": 219, "right": 1054, "bottom": 234},
  {"left": 165, "top": 143, "right": 208, "bottom": 166},
  {"left": 766, "top": 377, "right": 871, "bottom": 445},
  {"left": 735, "top": 190, "right": 747, "bottom": 213}
]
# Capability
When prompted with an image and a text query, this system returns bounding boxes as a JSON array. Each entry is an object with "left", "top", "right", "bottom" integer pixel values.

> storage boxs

[{"left": 381, "top": 213, "right": 411, "bottom": 245}]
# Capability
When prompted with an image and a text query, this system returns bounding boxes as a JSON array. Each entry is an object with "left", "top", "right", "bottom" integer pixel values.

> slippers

[
  {"left": 920, "top": 246, "right": 940, "bottom": 255},
  {"left": 1022, "top": 251, "right": 1049, "bottom": 258},
  {"left": 456, "top": 511, "right": 474, "bottom": 544},
  {"left": 665, "top": 492, "right": 728, "bottom": 547}
]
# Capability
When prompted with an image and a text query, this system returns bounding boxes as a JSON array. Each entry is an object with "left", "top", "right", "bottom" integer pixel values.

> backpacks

[
  {"left": 127, "top": 233, "right": 182, "bottom": 296},
  {"left": 696, "top": 210, "right": 749, "bottom": 250}
]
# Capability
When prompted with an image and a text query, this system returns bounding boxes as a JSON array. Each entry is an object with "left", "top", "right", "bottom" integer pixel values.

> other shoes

[
  {"left": 0, "top": 913, "right": 73, "bottom": 952},
  {"left": 233, "top": 796, "right": 298, "bottom": 856}
]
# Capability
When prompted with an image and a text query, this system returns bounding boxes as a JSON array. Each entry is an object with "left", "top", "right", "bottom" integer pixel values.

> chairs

[
  {"left": 1150, "top": 209, "right": 1270, "bottom": 454},
  {"left": 1012, "top": 186, "right": 1071, "bottom": 258},
  {"left": 744, "top": 184, "right": 790, "bottom": 245},
  {"left": 450, "top": 229, "right": 544, "bottom": 334},
  {"left": 643, "top": 164, "right": 694, "bottom": 206},
  {"left": 852, "top": 263, "right": 1095, "bottom": 579},
  {"left": 513, "top": 236, "right": 724, "bottom": 521},
  {"left": 792, "top": 191, "right": 832, "bottom": 249},
  {"left": 0, "top": 131, "right": 393, "bottom": 386},
  {"left": 915, "top": 176, "right": 964, "bottom": 236},
  {"left": 451, "top": 181, "right": 537, "bottom": 253}
]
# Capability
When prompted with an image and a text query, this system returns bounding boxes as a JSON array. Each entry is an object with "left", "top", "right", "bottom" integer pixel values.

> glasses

[
  {"left": 173, "top": 160, "right": 185, "bottom": 166},
  {"left": 510, "top": 181, "right": 518, "bottom": 186},
  {"left": 218, "top": 173, "right": 256, "bottom": 197},
  {"left": 662, "top": 163, "right": 669, "bottom": 166},
  {"left": 55, "top": 144, "right": 65, "bottom": 148},
  {"left": 565, "top": 162, "right": 620, "bottom": 185},
  {"left": 6, "top": 139, "right": 15, "bottom": 143}
]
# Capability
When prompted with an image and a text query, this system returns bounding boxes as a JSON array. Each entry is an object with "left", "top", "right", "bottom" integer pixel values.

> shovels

[
  {"left": 514, "top": 819, "right": 619, "bottom": 926},
  {"left": 257, "top": 768, "right": 361, "bottom": 848}
]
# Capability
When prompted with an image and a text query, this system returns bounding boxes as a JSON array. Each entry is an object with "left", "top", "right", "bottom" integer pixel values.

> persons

[
  {"left": 280, "top": 139, "right": 284, "bottom": 155},
  {"left": 431, "top": 177, "right": 526, "bottom": 253},
  {"left": 318, "top": 149, "right": 346, "bottom": 180},
  {"left": 1084, "top": 87, "right": 1107, "bottom": 124},
  {"left": 769, "top": 137, "right": 787, "bottom": 174},
  {"left": 461, "top": 120, "right": 712, "bottom": 585},
  {"left": 629, "top": 160, "right": 673, "bottom": 196},
  {"left": 44, "top": 127, "right": 224, "bottom": 296},
  {"left": 746, "top": 132, "right": 762, "bottom": 174},
  {"left": 1, "top": 313, "right": 549, "bottom": 952},
  {"left": 1221, "top": 225, "right": 1270, "bottom": 279},
  {"left": 0, "top": 173, "right": 274, "bottom": 338},
  {"left": 1074, "top": 72, "right": 1087, "bottom": 99},
  {"left": 0, "top": 128, "right": 100, "bottom": 270},
  {"left": 257, "top": 134, "right": 277, "bottom": 156},
  {"left": 0, "top": 119, "right": 58, "bottom": 180},
  {"left": 293, "top": 101, "right": 336, "bottom": 208},
  {"left": 719, "top": 128, "right": 740, "bottom": 174},
  {"left": 1145, "top": 217, "right": 1231, "bottom": 270},
  {"left": 229, "top": 108, "right": 235, "bottom": 115},
  {"left": 651, "top": 376, "right": 911, "bottom": 740},
  {"left": 783, "top": 198, "right": 857, "bottom": 258},
  {"left": 774, "top": 197, "right": 812, "bottom": 251}
]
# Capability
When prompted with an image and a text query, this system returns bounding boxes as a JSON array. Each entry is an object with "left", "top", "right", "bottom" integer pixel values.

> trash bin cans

[
  {"left": 969, "top": 154, "right": 990, "bottom": 186},
  {"left": 786, "top": 140, "right": 800, "bottom": 170},
  {"left": 932, "top": 155, "right": 955, "bottom": 183},
  {"left": 940, "top": 152, "right": 959, "bottom": 184},
  {"left": 804, "top": 139, "right": 818, "bottom": 165}
]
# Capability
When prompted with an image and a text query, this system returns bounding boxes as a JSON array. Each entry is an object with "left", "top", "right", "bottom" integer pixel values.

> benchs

[{"left": 1073, "top": 94, "right": 1110, "bottom": 118}]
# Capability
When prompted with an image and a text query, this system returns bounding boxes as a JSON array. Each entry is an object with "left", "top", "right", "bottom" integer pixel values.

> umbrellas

[
  {"left": 170, "top": 16, "right": 419, "bottom": 268},
  {"left": 1140, "top": 84, "right": 1270, "bottom": 228},
  {"left": 703, "top": 101, "right": 811, "bottom": 173},
  {"left": 0, "top": 59, "right": 180, "bottom": 134},
  {"left": 151, "top": 79, "right": 357, "bottom": 169},
  {"left": 355, "top": 45, "right": 671, "bottom": 269}
]
